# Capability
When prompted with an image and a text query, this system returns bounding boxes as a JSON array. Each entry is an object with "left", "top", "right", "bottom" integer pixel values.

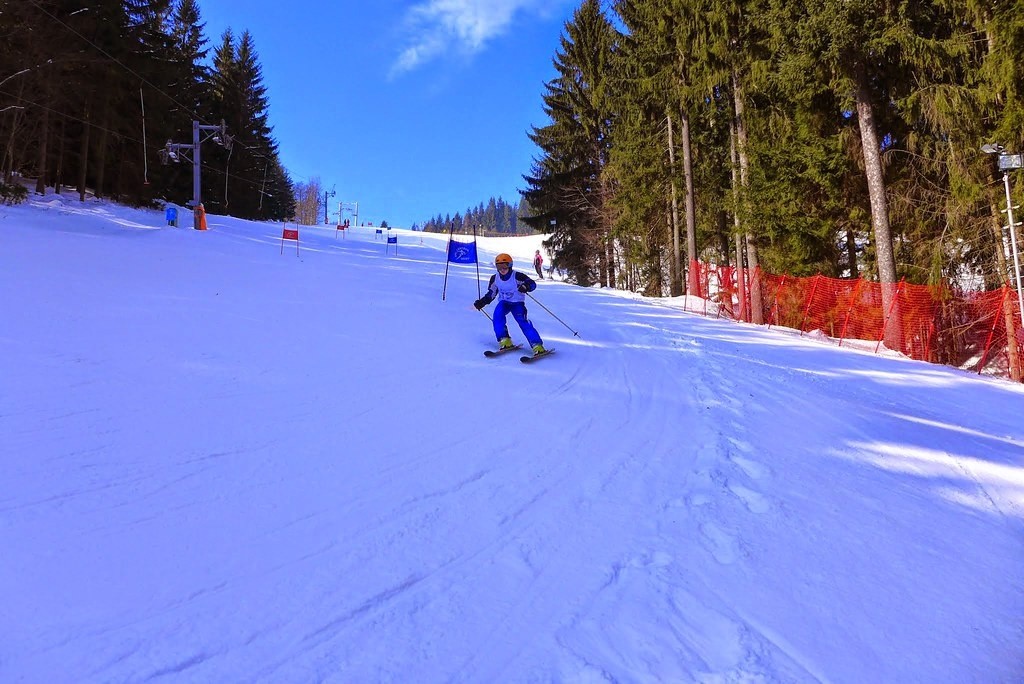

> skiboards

[{"left": 484, "top": 343, "right": 555, "bottom": 363}]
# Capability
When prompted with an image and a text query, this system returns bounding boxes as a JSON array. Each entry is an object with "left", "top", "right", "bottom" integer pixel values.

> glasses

[{"left": 496, "top": 262, "right": 512, "bottom": 269}]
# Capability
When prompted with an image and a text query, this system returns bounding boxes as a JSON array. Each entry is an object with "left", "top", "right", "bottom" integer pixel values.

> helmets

[{"left": 495, "top": 254, "right": 512, "bottom": 265}]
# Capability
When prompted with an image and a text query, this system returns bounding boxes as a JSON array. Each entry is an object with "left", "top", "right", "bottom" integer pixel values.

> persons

[
  {"left": 532, "top": 250, "right": 544, "bottom": 281},
  {"left": 473, "top": 253, "right": 549, "bottom": 358}
]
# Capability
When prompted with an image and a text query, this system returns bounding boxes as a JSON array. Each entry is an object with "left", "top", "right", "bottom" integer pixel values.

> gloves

[
  {"left": 473, "top": 299, "right": 485, "bottom": 311},
  {"left": 518, "top": 283, "right": 530, "bottom": 294}
]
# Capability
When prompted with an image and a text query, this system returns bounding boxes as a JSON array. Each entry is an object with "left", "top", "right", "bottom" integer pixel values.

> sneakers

[
  {"left": 532, "top": 343, "right": 548, "bottom": 357},
  {"left": 499, "top": 338, "right": 514, "bottom": 350}
]
